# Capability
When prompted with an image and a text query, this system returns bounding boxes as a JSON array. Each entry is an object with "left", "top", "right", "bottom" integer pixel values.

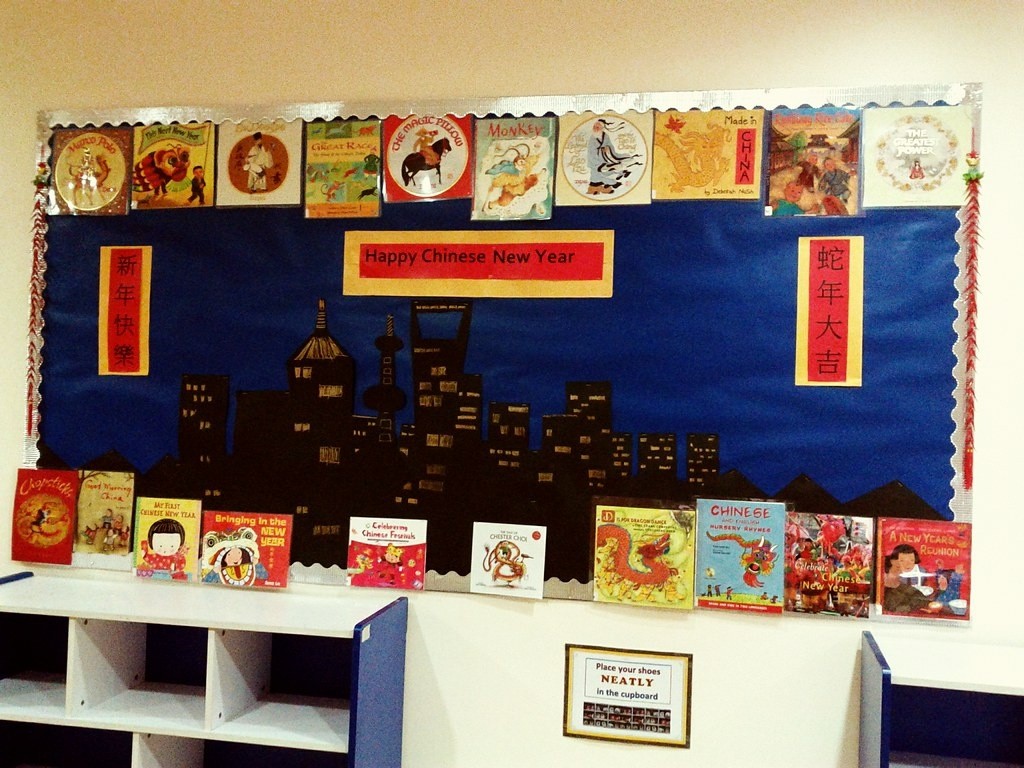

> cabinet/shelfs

[
  {"left": 858, "top": 631, "right": 1024, "bottom": 768},
  {"left": 0, "top": 572, "right": 408, "bottom": 768}
]
